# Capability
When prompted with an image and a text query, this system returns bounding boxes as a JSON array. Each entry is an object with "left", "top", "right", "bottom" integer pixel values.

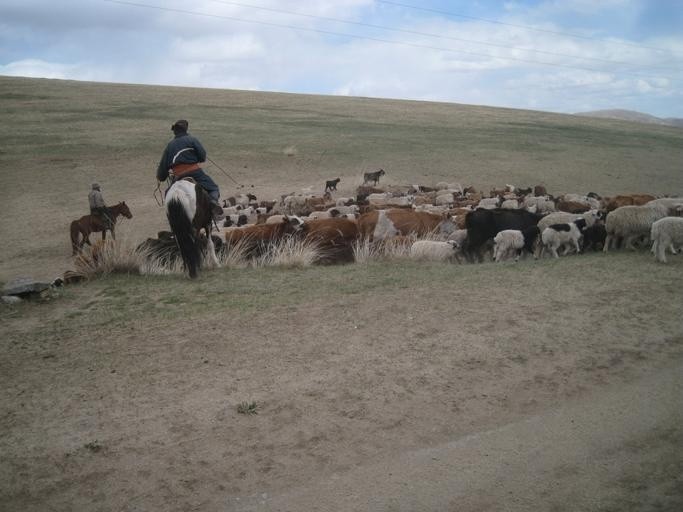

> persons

[
  {"left": 86, "top": 182, "right": 114, "bottom": 234},
  {"left": 154, "top": 119, "right": 224, "bottom": 217}
]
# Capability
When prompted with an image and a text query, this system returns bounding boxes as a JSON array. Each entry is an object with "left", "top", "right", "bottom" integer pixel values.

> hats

[
  {"left": 91, "top": 183, "right": 100, "bottom": 188},
  {"left": 171, "top": 120, "right": 188, "bottom": 135}
]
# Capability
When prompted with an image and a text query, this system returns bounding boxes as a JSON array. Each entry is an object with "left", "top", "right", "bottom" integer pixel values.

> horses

[
  {"left": 70, "top": 200, "right": 133, "bottom": 257},
  {"left": 164, "top": 168, "right": 222, "bottom": 281}
]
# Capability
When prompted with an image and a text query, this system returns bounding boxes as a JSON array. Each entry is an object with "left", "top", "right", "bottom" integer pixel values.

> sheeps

[
  {"left": 324, "top": 177, "right": 340, "bottom": 192},
  {"left": 362, "top": 168, "right": 386, "bottom": 186},
  {"left": 134, "top": 181, "right": 683, "bottom": 271}
]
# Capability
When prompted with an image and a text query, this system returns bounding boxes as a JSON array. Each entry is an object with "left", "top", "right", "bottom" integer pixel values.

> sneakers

[{"left": 209, "top": 199, "right": 223, "bottom": 215}]
